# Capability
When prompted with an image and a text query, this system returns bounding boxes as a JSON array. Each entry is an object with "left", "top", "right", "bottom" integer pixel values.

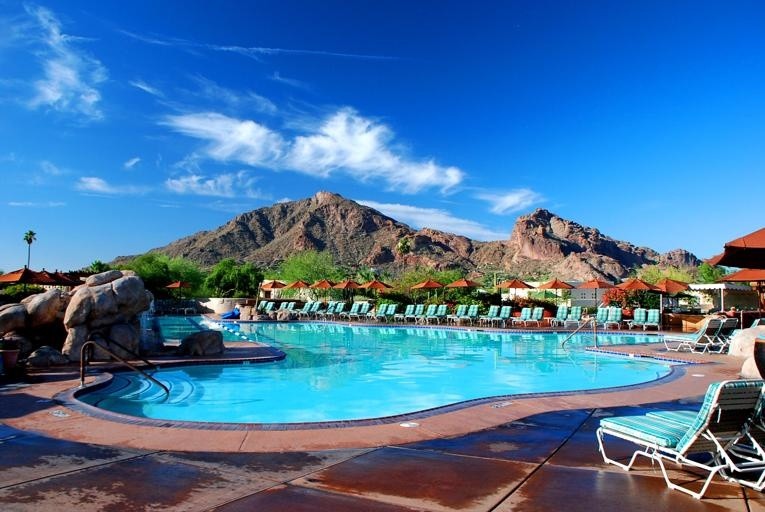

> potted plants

[{"left": 0, "top": 335, "right": 20, "bottom": 368}]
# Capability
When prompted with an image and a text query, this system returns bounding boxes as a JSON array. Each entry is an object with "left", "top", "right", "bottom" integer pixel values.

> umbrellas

[
  {"left": 0, "top": 265, "right": 76, "bottom": 289},
  {"left": 445, "top": 278, "right": 482, "bottom": 288},
  {"left": 495, "top": 278, "right": 533, "bottom": 297},
  {"left": 166, "top": 281, "right": 192, "bottom": 298},
  {"left": 537, "top": 277, "right": 689, "bottom": 306},
  {"left": 261, "top": 277, "right": 394, "bottom": 312},
  {"left": 411, "top": 278, "right": 442, "bottom": 299}
]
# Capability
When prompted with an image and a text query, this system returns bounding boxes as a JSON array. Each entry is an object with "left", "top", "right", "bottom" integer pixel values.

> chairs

[
  {"left": 366, "top": 304, "right": 387, "bottom": 322},
  {"left": 646, "top": 398, "right": 764, "bottom": 487},
  {"left": 480, "top": 305, "right": 500, "bottom": 327},
  {"left": 300, "top": 302, "right": 321, "bottom": 320},
  {"left": 405, "top": 305, "right": 424, "bottom": 323},
  {"left": 565, "top": 306, "right": 581, "bottom": 329},
  {"left": 415, "top": 305, "right": 437, "bottom": 324},
  {"left": 427, "top": 305, "right": 447, "bottom": 325},
  {"left": 663, "top": 319, "right": 723, "bottom": 353},
  {"left": 339, "top": 304, "right": 361, "bottom": 320},
  {"left": 683, "top": 318, "right": 737, "bottom": 354},
  {"left": 324, "top": 303, "right": 346, "bottom": 321},
  {"left": 512, "top": 308, "right": 532, "bottom": 327},
  {"left": 376, "top": 305, "right": 397, "bottom": 323},
  {"left": 257, "top": 301, "right": 313, "bottom": 317},
  {"left": 491, "top": 306, "right": 512, "bottom": 328},
  {"left": 550, "top": 306, "right": 567, "bottom": 328},
  {"left": 458, "top": 304, "right": 479, "bottom": 326},
  {"left": 629, "top": 308, "right": 646, "bottom": 330},
  {"left": 605, "top": 307, "right": 621, "bottom": 330},
  {"left": 157, "top": 299, "right": 197, "bottom": 315},
  {"left": 525, "top": 307, "right": 545, "bottom": 327},
  {"left": 349, "top": 304, "right": 371, "bottom": 321},
  {"left": 393, "top": 305, "right": 414, "bottom": 323},
  {"left": 643, "top": 309, "right": 660, "bottom": 331},
  {"left": 729, "top": 318, "right": 765, "bottom": 338},
  {"left": 446, "top": 305, "right": 468, "bottom": 324},
  {"left": 596, "top": 377, "right": 765, "bottom": 498},
  {"left": 590, "top": 309, "right": 608, "bottom": 330}
]
